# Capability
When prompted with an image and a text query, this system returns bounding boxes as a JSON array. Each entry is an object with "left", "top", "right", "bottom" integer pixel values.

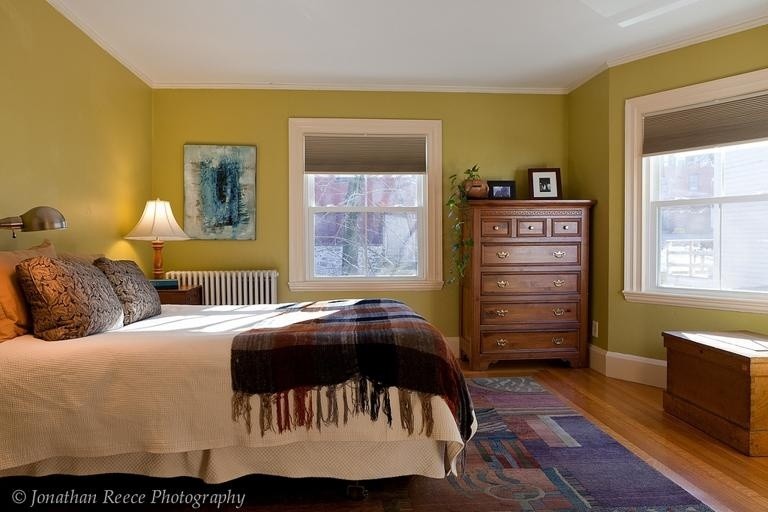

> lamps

[
  {"left": 0, "top": 202, "right": 69, "bottom": 243},
  {"left": 121, "top": 194, "right": 194, "bottom": 280}
]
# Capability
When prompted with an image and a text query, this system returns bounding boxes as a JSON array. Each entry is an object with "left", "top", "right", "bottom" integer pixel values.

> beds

[{"left": 0, "top": 295, "right": 482, "bottom": 487}]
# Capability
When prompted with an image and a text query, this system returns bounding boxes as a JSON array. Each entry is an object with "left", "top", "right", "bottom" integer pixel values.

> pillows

[
  {"left": 11, "top": 253, "right": 126, "bottom": 344},
  {"left": 57, "top": 249, "right": 106, "bottom": 265},
  {"left": 90, "top": 254, "right": 163, "bottom": 327},
  {"left": 0, "top": 236, "right": 63, "bottom": 345}
]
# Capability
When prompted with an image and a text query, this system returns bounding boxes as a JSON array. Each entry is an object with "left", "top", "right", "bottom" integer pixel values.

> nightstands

[{"left": 153, "top": 283, "right": 204, "bottom": 305}]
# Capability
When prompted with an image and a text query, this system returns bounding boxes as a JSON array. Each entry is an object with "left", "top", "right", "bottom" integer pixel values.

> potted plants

[{"left": 436, "top": 163, "right": 492, "bottom": 290}]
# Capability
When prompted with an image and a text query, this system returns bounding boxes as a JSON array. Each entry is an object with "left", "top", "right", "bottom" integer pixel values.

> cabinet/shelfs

[
  {"left": 450, "top": 195, "right": 597, "bottom": 375},
  {"left": 657, "top": 328, "right": 768, "bottom": 458}
]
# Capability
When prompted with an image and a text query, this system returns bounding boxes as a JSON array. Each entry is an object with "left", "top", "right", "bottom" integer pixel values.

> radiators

[{"left": 163, "top": 270, "right": 280, "bottom": 309}]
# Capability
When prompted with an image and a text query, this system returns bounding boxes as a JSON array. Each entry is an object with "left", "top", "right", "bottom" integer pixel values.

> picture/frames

[
  {"left": 528, "top": 167, "right": 563, "bottom": 200},
  {"left": 487, "top": 180, "right": 516, "bottom": 201}
]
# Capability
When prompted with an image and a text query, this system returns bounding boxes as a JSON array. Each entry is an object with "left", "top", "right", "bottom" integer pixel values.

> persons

[{"left": 542, "top": 181, "right": 550, "bottom": 191}]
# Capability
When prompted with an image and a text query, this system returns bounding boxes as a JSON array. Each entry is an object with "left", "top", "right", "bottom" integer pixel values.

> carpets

[{"left": 0, "top": 377, "right": 716, "bottom": 511}]
